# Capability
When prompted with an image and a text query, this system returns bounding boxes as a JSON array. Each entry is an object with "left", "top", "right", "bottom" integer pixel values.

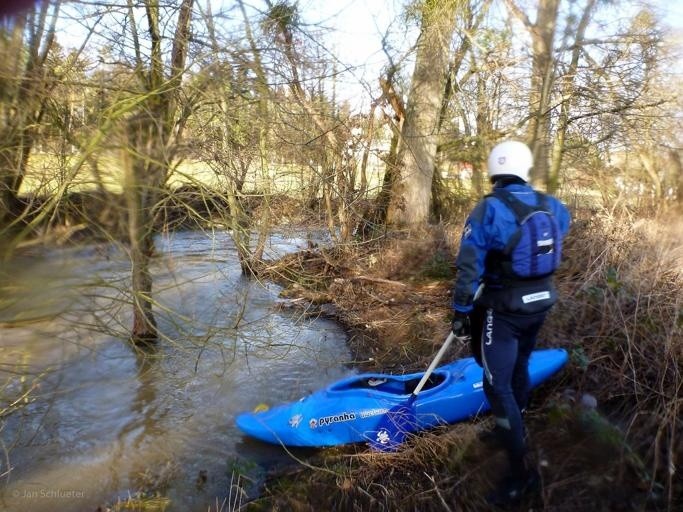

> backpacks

[{"left": 483, "top": 190, "right": 560, "bottom": 288}]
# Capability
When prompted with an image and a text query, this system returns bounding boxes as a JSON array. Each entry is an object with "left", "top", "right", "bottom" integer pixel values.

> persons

[{"left": 452, "top": 140, "right": 572, "bottom": 512}]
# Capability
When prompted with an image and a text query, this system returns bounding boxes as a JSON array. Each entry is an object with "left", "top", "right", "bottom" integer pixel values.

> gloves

[{"left": 449, "top": 309, "right": 472, "bottom": 337}]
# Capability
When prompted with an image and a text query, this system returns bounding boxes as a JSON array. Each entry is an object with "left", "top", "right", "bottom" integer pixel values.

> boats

[{"left": 233, "top": 346, "right": 571, "bottom": 448}]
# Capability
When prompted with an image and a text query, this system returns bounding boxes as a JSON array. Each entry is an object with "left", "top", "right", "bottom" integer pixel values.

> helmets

[{"left": 488, "top": 141, "right": 535, "bottom": 182}]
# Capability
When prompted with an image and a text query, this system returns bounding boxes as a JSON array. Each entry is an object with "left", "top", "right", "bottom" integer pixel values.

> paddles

[{"left": 368, "top": 285, "right": 482, "bottom": 450}]
